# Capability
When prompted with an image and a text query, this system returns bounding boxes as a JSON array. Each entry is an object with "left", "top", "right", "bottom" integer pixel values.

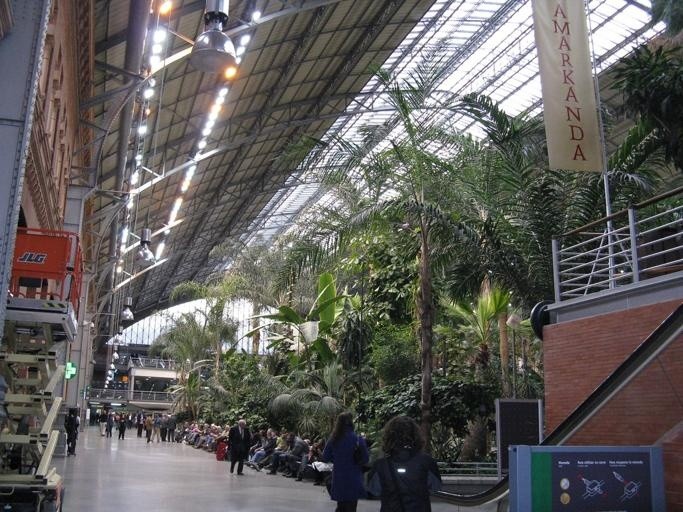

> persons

[
  {"left": 63, "top": 408, "right": 80, "bottom": 456},
  {"left": 321, "top": 411, "right": 368, "bottom": 512},
  {"left": 366, "top": 414, "right": 440, "bottom": 512},
  {"left": 93, "top": 407, "right": 326, "bottom": 486}
]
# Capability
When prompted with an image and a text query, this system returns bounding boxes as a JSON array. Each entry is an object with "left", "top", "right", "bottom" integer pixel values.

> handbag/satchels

[{"left": 354, "top": 446, "right": 364, "bottom": 465}]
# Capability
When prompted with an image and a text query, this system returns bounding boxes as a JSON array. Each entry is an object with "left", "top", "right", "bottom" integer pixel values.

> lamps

[
  {"left": 188, "top": 0, "right": 237, "bottom": 73},
  {"left": 132, "top": 7, "right": 173, "bottom": 267},
  {"left": 119, "top": 97, "right": 150, "bottom": 322}
]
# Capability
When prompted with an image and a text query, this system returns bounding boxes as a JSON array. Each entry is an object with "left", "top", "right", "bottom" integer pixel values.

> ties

[{"left": 241, "top": 428, "right": 243, "bottom": 438}]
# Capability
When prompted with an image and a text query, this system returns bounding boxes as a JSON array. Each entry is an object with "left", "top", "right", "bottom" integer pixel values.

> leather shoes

[
  {"left": 237, "top": 471, "right": 242, "bottom": 474},
  {"left": 313, "top": 479, "right": 320, "bottom": 486},
  {"left": 230, "top": 468, "right": 234, "bottom": 472},
  {"left": 246, "top": 461, "right": 303, "bottom": 482}
]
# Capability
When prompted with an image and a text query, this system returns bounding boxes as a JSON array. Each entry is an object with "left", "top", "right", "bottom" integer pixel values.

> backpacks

[{"left": 66, "top": 417, "right": 78, "bottom": 432}]
